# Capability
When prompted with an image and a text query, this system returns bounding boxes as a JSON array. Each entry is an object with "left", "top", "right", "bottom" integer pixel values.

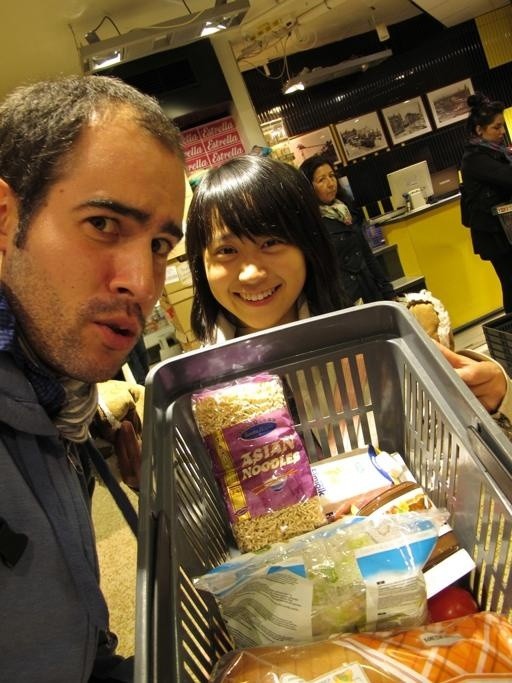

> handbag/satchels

[{"left": 458, "top": 182, "right": 475, "bottom": 228}]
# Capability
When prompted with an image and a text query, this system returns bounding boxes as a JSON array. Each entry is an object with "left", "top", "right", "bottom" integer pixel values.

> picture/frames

[
  {"left": 381, "top": 95, "right": 433, "bottom": 145},
  {"left": 333, "top": 111, "right": 389, "bottom": 162},
  {"left": 426, "top": 79, "right": 475, "bottom": 128},
  {"left": 270, "top": 127, "right": 342, "bottom": 184}
]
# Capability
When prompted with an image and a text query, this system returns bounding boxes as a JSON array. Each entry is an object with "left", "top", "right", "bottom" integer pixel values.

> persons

[
  {"left": 460, "top": 92, "right": 512, "bottom": 318},
  {"left": 87, "top": 410, "right": 143, "bottom": 493},
  {"left": 0, "top": 70, "right": 187, "bottom": 683},
  {"left": 183, "top": 153, "right": 511, "bottom": 507},
  {"left": 298, "top": 155, "right": 406, "bottom": 308}
]
